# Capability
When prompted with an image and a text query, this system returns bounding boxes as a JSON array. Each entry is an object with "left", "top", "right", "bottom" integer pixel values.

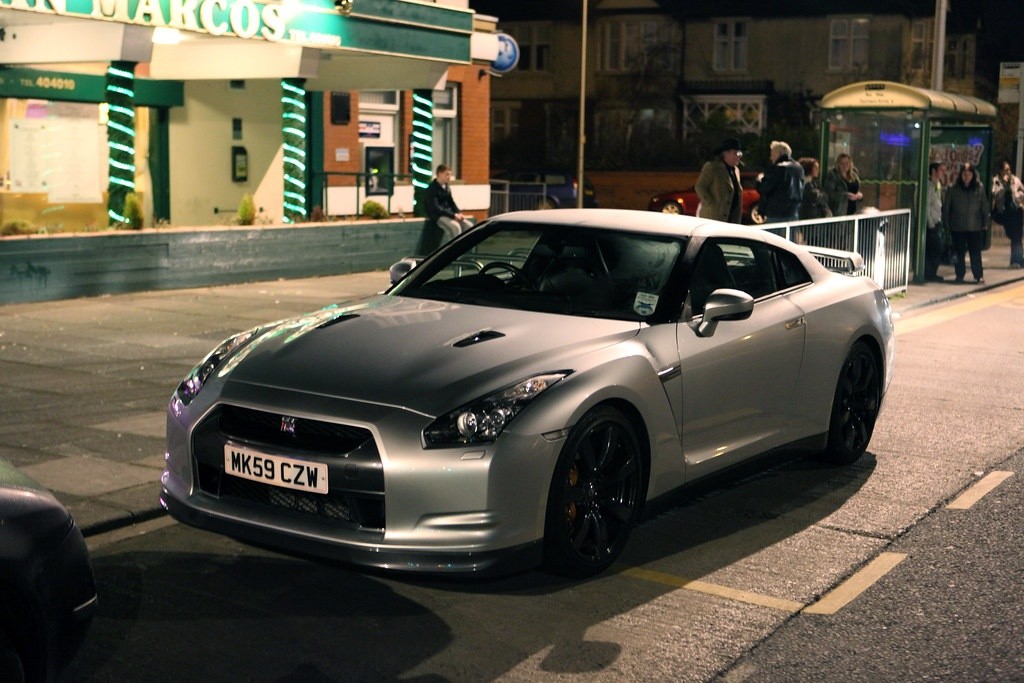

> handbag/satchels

[
  {"left": 992, "top": 203, "right": 1008, "bottom": 226},
  {"left": 931, "top": 229, "right": 961, "bottom": 265}
]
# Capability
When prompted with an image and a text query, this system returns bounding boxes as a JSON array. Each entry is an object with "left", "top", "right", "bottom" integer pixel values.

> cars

[
  {"left": 158, "top": 209, "right": 896, "bottom": 581},
  {"left": 648, "top": 170, "right": 769, "bottom": 226},
  {"left": 488, "top": 161, "right": 599, "bottom": 218}
]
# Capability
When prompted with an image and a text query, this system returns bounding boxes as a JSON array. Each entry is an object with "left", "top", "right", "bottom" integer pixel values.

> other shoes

[
  {"left": 977, "top": 278, "right": 984, "bottom": 283},
  {"left": 956, "top": 277, "right": 963, "bottom": 281}
]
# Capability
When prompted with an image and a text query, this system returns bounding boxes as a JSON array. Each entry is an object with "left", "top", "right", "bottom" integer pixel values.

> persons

[
  {"left": 426, "top": 164, "right": 474, "bottom": 242},
  {"left": 695, "top": 140, "right": 748, "bottom": 224},
  {"left": 945, "top": 161, "right": 992, "bottom": 284},
  {"left": 926, "top": 160, "right": 945, "bottom": 283},
  {"left": 756, "top": 140, "right": 864, "bottom": 270},
  {"left": 992, "top": 157, "right": 1024, "bottom": 270}
]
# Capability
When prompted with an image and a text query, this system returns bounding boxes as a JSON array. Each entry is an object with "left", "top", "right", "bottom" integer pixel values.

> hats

[{"left": 713, "top": 138, "right": 749, "bottom": 156}]
